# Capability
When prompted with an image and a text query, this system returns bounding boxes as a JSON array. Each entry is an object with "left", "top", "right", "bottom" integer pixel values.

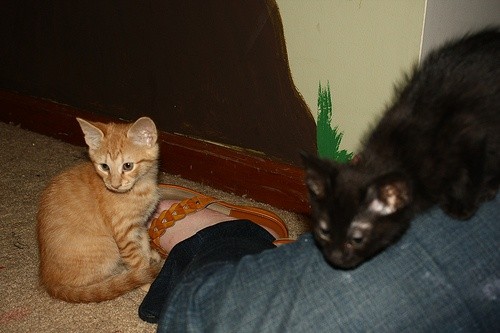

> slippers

[{"left": 144, "top": 183, "right": 288, "bottom": 259}]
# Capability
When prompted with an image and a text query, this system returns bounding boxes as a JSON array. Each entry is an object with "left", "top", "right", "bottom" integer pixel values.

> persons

[{"left": 139, "top": 185, "right": 500, "bottom": 333}]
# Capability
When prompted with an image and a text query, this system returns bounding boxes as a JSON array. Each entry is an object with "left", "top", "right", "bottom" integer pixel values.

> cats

[
  {"left": 36, "top": 115, "right": 168, "bottom": 303},
  {"left": 298, "top": 24, "right": 500, "bottom": 271}
]
